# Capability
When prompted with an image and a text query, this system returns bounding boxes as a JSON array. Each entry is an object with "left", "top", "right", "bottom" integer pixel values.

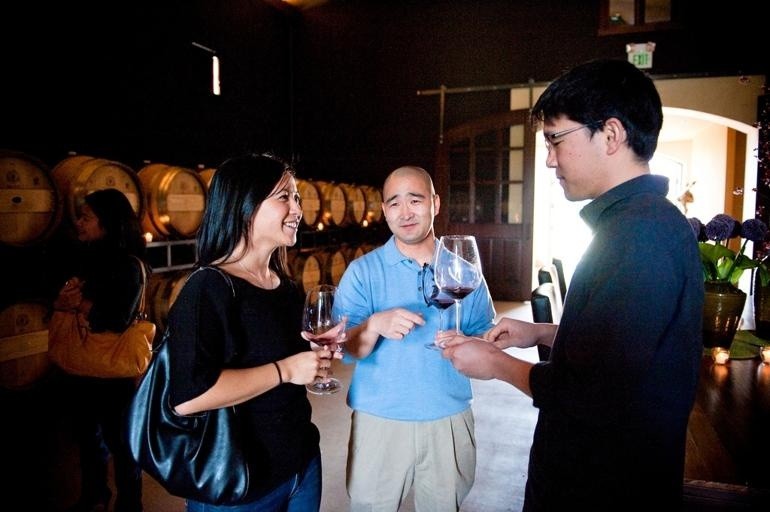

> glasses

[
  {"left": 422, "top": 264, "right": 441, "bottom": 307},
  {"left": 545, "top": 120, "right": 602, "bottom": 149}
]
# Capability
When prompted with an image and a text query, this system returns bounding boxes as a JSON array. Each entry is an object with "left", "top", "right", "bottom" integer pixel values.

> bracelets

[{"left": 272, "top": 359, "right": 286, "bottom": 386}]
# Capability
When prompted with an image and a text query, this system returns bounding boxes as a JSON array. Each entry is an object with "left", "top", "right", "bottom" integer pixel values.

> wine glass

[
  {"left": 424, "top": 262, "right": 455, "bottom": 354},
  {"left": 302, "top": 285, "right": 343, "bottom": 396},
  {"left": 434, "top": 234, "right": 483, "bottom": 336}
]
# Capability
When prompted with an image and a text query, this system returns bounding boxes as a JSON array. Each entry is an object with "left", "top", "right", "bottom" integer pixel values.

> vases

[{"left": 698, "top": 287, "right": 750, "bottom": 349}]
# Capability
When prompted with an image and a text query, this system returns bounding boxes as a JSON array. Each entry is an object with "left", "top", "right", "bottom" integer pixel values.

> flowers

[{"left": 684, "top": 214, "right": 769, "bottom": 283}]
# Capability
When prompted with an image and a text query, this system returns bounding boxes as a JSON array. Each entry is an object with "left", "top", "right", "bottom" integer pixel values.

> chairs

[
  {"left": 530, "top": 284, "right": 573, "bottom": 364},
  {"left": 554, "top": 253, "right": 567, "bottom": 298},
  {"left": 537, "top": 265, "right": 561, "bottom": 296}
]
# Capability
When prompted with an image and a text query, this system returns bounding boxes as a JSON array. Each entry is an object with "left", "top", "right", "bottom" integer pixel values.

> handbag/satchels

[
  {"left": 126, "top": 268, "right": 255, "bottom": 505},
  {"left": 47, "top": 257, "right": 156, "bottom": 379}
]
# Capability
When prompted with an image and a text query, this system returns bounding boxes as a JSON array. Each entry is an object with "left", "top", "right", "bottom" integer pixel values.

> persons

[
  {"left": 166, "top": 154, "right": 348, "bottom": 512},
  {"left": 442, "top": 60, "right": 702, "bottom": 512},
  {"left": 52, "top": 189, "right": 148, "bottom": 512},
  {"left": 330, "top": 166, "right": 495, "bottom": 512}
]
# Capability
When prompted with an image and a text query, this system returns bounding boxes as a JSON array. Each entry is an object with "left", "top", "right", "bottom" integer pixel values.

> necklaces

[{"left": 230, "top": 253, "right": 275, "bottom": 290}]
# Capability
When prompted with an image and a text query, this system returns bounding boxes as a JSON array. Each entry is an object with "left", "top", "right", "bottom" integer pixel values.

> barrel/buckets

[
  {"left": 295, "top": 177, "right": 321, "bottom": 227},
  {"left": 136, "top": 163, "right": 209, "bottom": 240},
  {"left": 0, "top": 156, "right": 65, "bottom": 248},
  {"left": 145, "top": 271, "right": 193, "bottom": 335},
  {"left": 338, "top": 183, "right": 367, "bottom": 225},
  {"left": 312, "top": 246, "right": 348, "bottom": 287},
  {"left": 358, "top": 185, "right": 384, "bottom": 224},
  {"left": 286, "top": 251, "right": 321, "bottom": 304},
  {"left": 341, "top": 242, "right": 364, "bottom": 266},
  {"left": 200, "top": 169, "right": 217, "bottom": 192},
  {"left": 360, "top": 243, "right": 375, "bottom": 253},
  {"left": 376, "top": 242, "right": 383, "bottom": 247},
  {"left": 48, "top": 155, "right": 146, "bottom": 231},
  {"left": 0, "top": 300, "right": 52, "bottom": 393},
  {"left": 311, "top": 180, "right": 348, "bottom": 228}
]
